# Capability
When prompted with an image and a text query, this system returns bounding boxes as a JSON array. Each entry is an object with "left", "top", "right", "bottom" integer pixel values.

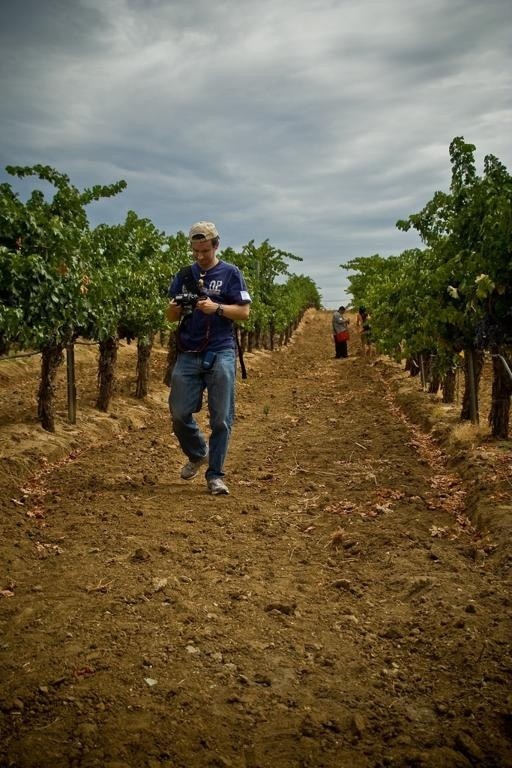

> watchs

[{"left": 216, "top": 303, "right": 223, "bottom": 317}]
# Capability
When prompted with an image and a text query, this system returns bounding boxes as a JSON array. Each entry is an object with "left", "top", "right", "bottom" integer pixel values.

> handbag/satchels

[{"left": 334, "top": 330, "right": 349, "bottom": 343}]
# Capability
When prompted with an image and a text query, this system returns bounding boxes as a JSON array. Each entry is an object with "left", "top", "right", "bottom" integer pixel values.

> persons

[
  {"left": 357, "top": 306, "right": 372, "bottom": 357},
  {"left": 164, "top": 221, "right": 253, "bottom": 496},
  {"left": 332, "top": 306, "right": 350, "bottom": 358}
]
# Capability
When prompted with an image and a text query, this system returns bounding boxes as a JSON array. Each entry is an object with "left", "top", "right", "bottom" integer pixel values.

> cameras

[{"left": 174, "top": 294, "right": 198, "bottom": 315}]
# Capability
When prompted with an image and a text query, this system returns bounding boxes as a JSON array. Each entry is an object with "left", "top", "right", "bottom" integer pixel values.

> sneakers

[
  {"left": 207, "top": 477, "right": 229, "bottom": 495},
  {"left": 181, "top": 445, "right": 210, "bottom": 479}
]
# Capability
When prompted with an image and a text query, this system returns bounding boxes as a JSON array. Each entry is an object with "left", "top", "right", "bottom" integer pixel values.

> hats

[{"left": 188, "top": 221, "right": 221, "bottom": 243}]
